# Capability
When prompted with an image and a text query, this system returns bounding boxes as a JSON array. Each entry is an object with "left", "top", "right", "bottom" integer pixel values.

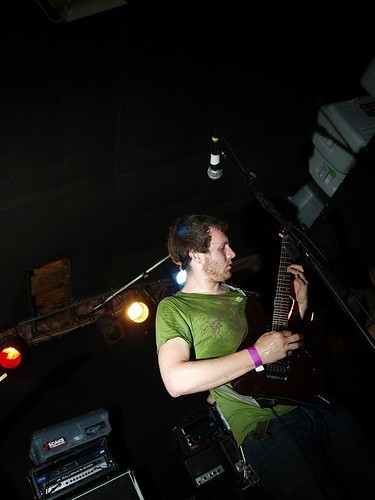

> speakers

[{"left": 184, "top": 435, "right": 243, "bottom": 500}]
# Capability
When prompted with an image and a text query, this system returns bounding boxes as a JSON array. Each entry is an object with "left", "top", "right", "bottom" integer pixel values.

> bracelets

[{"left": 246, "top": 345, "right": 265, "bottom": 372}]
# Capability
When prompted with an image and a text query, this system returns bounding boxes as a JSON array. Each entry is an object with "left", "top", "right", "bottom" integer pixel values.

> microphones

[{"left": 207, "top": 127, "right": 224, "bottom": 180}]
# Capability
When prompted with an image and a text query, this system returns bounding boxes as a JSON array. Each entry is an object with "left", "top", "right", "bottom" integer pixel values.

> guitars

[{"left": 231, "top": 223, "right": 310, "bottom": 407}]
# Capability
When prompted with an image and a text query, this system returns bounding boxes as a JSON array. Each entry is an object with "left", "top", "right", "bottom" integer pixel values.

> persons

[{"left": 155, "top": 214, "right": 375, "bottom": 500}]
